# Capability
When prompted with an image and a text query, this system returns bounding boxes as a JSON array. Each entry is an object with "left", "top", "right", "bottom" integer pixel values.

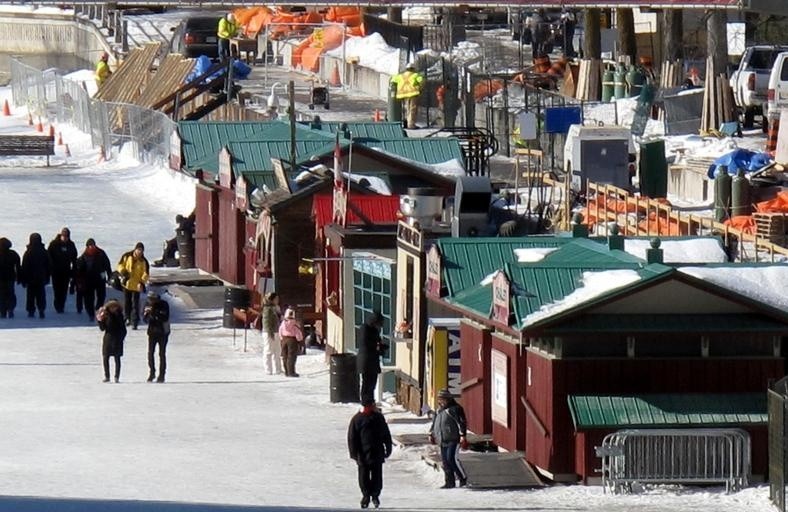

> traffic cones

[
  {"left": 2, "top": 99, "right": 11, "bottom": 116},
  {"left": 24, "top": 109, "right": 72, "bottom": 159}
]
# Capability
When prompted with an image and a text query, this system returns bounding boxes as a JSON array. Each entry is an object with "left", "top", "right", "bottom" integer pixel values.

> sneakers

[
  {"left": 358, "top": 497, "right": 371, "bottom": 509},
  {"left": 147, "top": 375, "right": 157, "bottom": 382},
  {"left": 459, "top": 476, "right": 467, "bottom": 487},
  {"left": 114, "top": 376, "right": 120, "bottom": 383},
  {"left": 2, "top": 308, "right": 96, "bottom": 322},
  {"left": 125, "top": 320, "right": 139, "bottom": 331},
  {"left": 103, "top": 377, "right": 111, "bottom": 382},
  {"left": 371, "top": 496, "right": 380, "bottom": 508},
  {"left": 265, "top": 370, "right": 300, "bottom": 379},
  {"left": 439, "top": 479, "right": 456, "bottom": 489},
  {"left": 156, "top": 374, "right": 166, "bottom": 383}
]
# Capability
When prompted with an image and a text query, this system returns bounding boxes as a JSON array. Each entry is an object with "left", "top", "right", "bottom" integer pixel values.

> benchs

[{"left": 0, "top": 135, "right": 56, "bottom": 167}]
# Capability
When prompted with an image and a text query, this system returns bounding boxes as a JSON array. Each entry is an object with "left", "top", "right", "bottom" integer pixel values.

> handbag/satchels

[
  {"left": 110, "top": 272, "right": 123, "bottom": 292},
  {"left": 376, "top": 342, "right": 387, "bottom": 358}
]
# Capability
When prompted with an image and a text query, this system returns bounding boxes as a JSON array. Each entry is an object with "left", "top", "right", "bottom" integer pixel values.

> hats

[
  {"left": 0, "top": 228, "right": 96, "bottom": 248},
  {"left": 436, "top": 389, "right": 454, "bottom": 398},
  {"left": 145, "top": 290, "right": 159, "bottom": 299},
  {"left": 282, "top": 308, "right": 296, "bottom": 319},
  {"left": 361, "top": 395, "right": 377, "bottom": 406},
  {"left": 134, "top": 242, "right": 144, "bottom": 250}
]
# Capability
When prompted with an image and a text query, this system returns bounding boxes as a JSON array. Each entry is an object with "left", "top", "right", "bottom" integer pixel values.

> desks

[{"left": 229, "top": 36, "right": 258, "bottom": 67}]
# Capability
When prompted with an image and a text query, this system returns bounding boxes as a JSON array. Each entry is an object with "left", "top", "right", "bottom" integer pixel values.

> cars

[
  {"left": 678, "top": 46, "right": 788, "bottom": 131},
  {"left": 166, "top": 15, "right": 230, "bottom": 61},
  {"left": 510, "top": 12, "right": 565, "bottom": 54}
]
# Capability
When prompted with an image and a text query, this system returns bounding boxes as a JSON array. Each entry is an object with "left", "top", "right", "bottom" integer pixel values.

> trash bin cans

[
  {"left": 330, "top": 353, "right": 361, "bottom": 403},
  {"left": 223, "top": 286, "right": 251, "bottom": 329},
  {"left": 175, "top": 228, "right": 195, "bottom": 269}
]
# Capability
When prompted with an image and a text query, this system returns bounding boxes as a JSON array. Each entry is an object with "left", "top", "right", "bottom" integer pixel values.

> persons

[
  {"left": 628, "top": 153, "right": 637, "bottom": 185},
  {"left": 0, "top": 215, "right": 196, "bottom": 382},
  {"left": 429, "top": 388, "right": 467, "bottom": 488},
  {"left": 357, "top": 312, "right": 389, "bottom": 412},
  {"left": 390, "top": 63, "right": 427, "bottom": 129},
  {"left": 348, "top": 396, "right": 392, "bottom": 508},
  {"left": 513, "top": 112, "right": 544, "bottom": 149},
  {"left": 217, "top": 13, "right": 236, "bottom": 59},
  {"left": 261, "top": 292, "right": 281, "bottom": 375},
  {"left": 95, "top": 51, "right": 112, "bottom": 90},
  {"left": 436, "top": 78, "right": 462, "bottom": 129},
  {"left": 525, "top": 8, "right": 545, "bottom": 58},
  {"left": 279, "top": 308, "right": 303, "bottom": 377}
]
